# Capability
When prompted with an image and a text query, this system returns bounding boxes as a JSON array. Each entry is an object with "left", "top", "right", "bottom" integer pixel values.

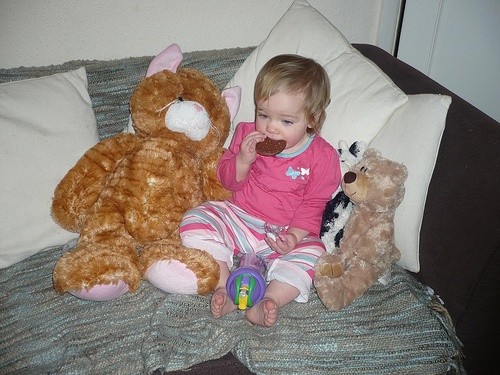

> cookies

[{"left": 255, "top": 138, "right": 286, "bottom": 155}]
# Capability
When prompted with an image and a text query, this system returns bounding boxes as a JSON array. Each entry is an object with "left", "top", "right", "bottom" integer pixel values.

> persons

[{"left": 178, "top": 54, "right": 341, "bottom": 328}]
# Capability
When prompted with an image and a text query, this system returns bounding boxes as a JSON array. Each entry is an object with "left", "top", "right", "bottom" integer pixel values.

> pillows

[
  {"left": 220, "top": 0, "right": 408, "bottom": 154},
  {"left": 367, "top": 92, "right": 453, "bottom": 273},
  {"left": 0, "top": 65, "right": 100, "bottom": 271}
]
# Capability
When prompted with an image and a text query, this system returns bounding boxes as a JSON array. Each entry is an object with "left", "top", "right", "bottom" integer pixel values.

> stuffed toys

[
  {"left": 50, "top": 43, "right": 242, "bottom": 303},
  {"left": 313, "top": 148, "right": 408, "bottom": 312},
  {"left": 319, "top": 140, "right": 366, "bottom": 254}
]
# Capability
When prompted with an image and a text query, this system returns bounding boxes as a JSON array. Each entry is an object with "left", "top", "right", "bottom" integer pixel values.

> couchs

[{"left": 0, "top": 43, "right": 500, "bottom": 375}]
